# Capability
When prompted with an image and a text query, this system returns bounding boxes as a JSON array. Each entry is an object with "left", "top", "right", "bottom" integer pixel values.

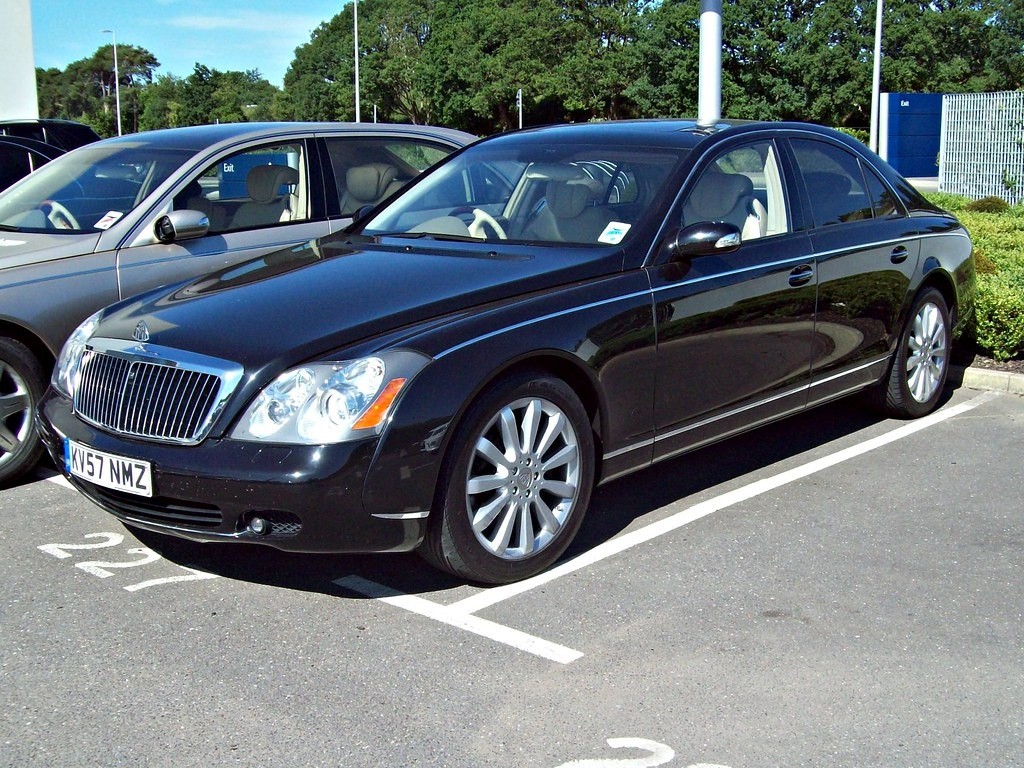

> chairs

[
  {"left": 228, "top": 165, "right": 299, "bottom": 229},
  {"left": 516, "top": 178, "right": 628, "bottom": 245},
  {"left": 180, "top": 182, "right": 228, "bottom": 231},
  {"left": 680, "top": 172, "right": 767, "bottom": 241},
  {"left": 340, "top": 163, "right": 407, "bottom": 215},
  {"left": 803, "top": 171, "right": 851, "bottom": 225}
]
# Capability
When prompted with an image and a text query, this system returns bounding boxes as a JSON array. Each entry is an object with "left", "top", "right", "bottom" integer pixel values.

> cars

[
  {"left": 0, "top": 122, "right": 546, "bottom": 488},
  {"left": 0, "top": 136, "right": 137, "bottom": 222},
  {"left": 34, "top": 119, "right": 977, "bottom": 589},
  {"left": 0, "top": 119, "right": 137, "bottom": 180}
]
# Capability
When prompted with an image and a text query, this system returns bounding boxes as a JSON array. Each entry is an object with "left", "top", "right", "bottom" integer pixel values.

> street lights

[{"left": 102, "top": 29, "right": 122, "bottom": 137}]
